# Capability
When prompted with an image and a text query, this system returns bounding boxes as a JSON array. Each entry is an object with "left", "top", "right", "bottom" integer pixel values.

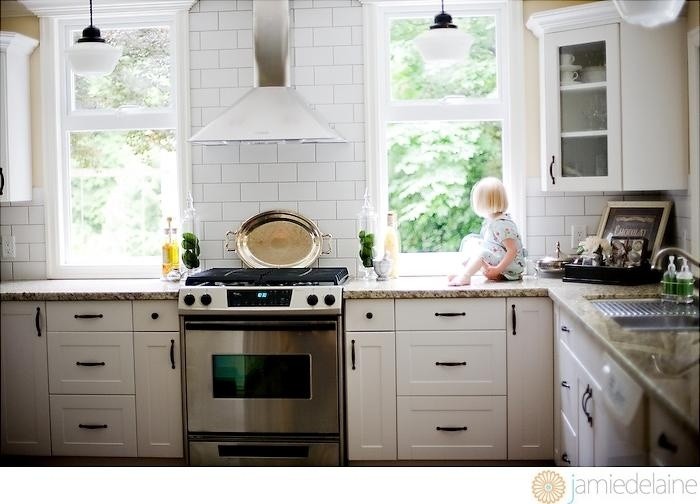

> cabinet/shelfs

[
  {"left": 0, "top": 31, "right": 39, "bottom": 203},
  {"left": 524, "top": 0, "right": 688, "bottom": 195},
  {"left": 554, "top": 300, "right": 579, "bottom": 467},
  {"left": 132, "top": 296, "right": 192, "bottom": 461},
  {"left": 344, "top": 299, "right": 397, "bottom": 461},
  {"left": 650, "top": 394, "right": 700, "bottom": 466},
  {"left": 579, "top": 330, "right": 649, "bottom": 466},
  {"left": 1, "top": 300, "right": 52, "bottom": 459},
  {"left": 45, "top": 300, "right": 138, "bottom": 459},
  {"left": 394, "top": 298, "right": 509, "bottom": 462},
  {"left": 506, "top": 297, "right": 554, "bottom": 463}
]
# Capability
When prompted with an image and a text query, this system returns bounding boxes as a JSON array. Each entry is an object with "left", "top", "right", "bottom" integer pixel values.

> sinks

[{"left": 610, "top": 313, "right": 700, "bottom": 333}]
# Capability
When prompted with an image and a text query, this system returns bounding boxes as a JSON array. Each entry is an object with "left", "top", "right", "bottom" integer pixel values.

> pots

[{"left": 223, "top": 208, "right": 332, "bottom": 267}]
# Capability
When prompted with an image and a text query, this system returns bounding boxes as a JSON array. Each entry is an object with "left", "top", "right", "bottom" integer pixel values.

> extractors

[{"left": 187, "top": 0, "right": 349, "bottom": 143}]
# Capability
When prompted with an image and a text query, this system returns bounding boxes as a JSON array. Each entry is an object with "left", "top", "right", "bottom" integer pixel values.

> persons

[{"left": 443, "top": 177, "right": 524, "bottom": 286}]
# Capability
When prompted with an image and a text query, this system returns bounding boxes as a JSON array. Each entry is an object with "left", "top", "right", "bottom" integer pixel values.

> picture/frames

[{"left": 596, "top": 200, "right": 673, "bottom": 265}]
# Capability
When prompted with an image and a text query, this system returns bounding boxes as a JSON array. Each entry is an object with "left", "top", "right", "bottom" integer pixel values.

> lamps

[
  {"left": 66, "top": 2, "right": 123, "bottom": 78},
  {"left": 615, "top": 1, "right": 685, "bottom": 31},
  {"left": 414, "top": 0, "right": 474, "bottom": 64}
]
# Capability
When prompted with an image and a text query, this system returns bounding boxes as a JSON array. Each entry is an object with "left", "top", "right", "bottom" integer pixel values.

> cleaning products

[{"left": 163, "top": 217, "right": 180, "bottom": 276}]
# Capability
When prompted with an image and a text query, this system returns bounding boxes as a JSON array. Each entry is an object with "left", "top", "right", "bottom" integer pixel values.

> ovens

[{"left": 182, "top": 321, "right": 344, "bottom": 466}]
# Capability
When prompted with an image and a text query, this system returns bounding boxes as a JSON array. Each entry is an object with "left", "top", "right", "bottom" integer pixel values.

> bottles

[
  {"left": 356, "top": 185, "right": 379, "bottom": 279},
  {"left": 161, "top": 216, "right": 181, "bottom": 282},
  {"left": 383, "top": 211, "right": 399, "bottom": 279},
  {"left": 182, "top": 192, "right": 200, "bottom": 278}
]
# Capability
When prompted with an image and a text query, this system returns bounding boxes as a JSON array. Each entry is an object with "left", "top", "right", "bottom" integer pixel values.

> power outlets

[
  {"left": 570, "top": 224, "right": 587, "bottom": 251},
  {"left": 2, "top": 235, "right": 16, "bottom": 257}
]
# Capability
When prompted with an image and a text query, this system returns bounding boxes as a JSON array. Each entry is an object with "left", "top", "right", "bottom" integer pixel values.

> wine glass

[{"left": 575, "top": 92, "right": 606, "bottom": 130}]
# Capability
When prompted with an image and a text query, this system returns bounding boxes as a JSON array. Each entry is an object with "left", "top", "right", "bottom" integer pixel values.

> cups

[
  {"left": 561, "top": 52, "right": 606, "bottom": 86},
  {"left": 373, "top": 258, "right": 394, "bottom": 281}
]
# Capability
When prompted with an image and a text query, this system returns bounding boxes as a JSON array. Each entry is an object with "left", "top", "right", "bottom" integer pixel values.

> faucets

[{"left": 651, "top": 247, "right": 700, "bottom": 271}]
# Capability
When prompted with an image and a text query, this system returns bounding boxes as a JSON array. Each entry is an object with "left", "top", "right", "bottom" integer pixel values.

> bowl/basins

[{"left": 532, "top": 254, "right": 576, "bottom": 279}]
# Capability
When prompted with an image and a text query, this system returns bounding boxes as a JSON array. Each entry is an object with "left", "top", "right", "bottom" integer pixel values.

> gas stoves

[{"left": 178, "top": 267, "right": 349, "bottom": 316}]
